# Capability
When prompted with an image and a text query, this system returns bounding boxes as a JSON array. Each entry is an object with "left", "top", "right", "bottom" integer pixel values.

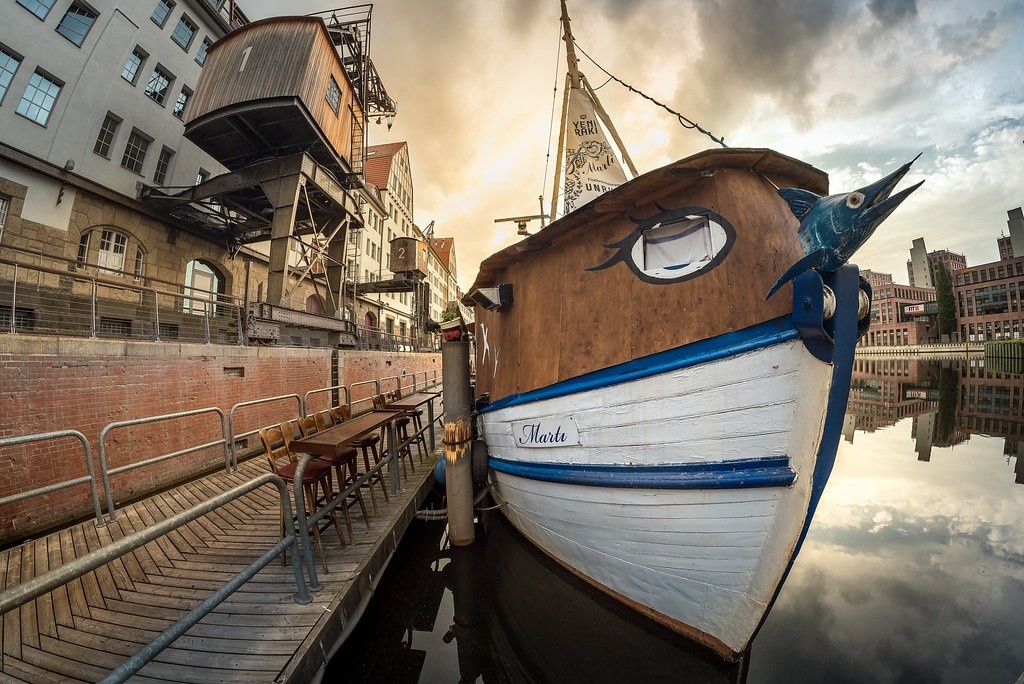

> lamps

[
  {"left": 65, "top": 159, "right": 75, "bottom": 170},
  {"left": 470, "top": 284, "right": 513, "bottom": 311}
]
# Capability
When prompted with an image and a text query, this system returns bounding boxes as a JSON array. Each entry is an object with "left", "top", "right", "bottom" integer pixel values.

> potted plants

[{"left": 439, "top": 307, "right": 462, "bottom": 342}]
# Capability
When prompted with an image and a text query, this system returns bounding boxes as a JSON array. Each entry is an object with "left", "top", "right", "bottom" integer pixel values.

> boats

[
  {"left": 434, "top": 492, "right": 755, "bottom": 684},
  {"left": 459, "top": 1, "right": 927, "bottom": 665}
]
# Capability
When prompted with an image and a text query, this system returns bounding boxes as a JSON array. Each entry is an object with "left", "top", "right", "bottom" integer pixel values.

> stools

[
  {"left": 330, "top": 404, "right": 390, "bottom": 518},
  {"left": 259, "top": 421, "right": 348, "bottom": 575},
  {"left": 298, "top": 412, "right": 371, "bottom": 545},
  {"left": 372, "top": 389, "right": 428, "bottom": 482}
]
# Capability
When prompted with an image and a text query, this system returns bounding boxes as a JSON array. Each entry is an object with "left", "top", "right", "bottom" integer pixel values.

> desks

[
  {"left": 289, "top": 409, "right": 404, "bottom": 593},
  {"left": 387, "top": 391, "right": 440, "bottom": 492}
]
